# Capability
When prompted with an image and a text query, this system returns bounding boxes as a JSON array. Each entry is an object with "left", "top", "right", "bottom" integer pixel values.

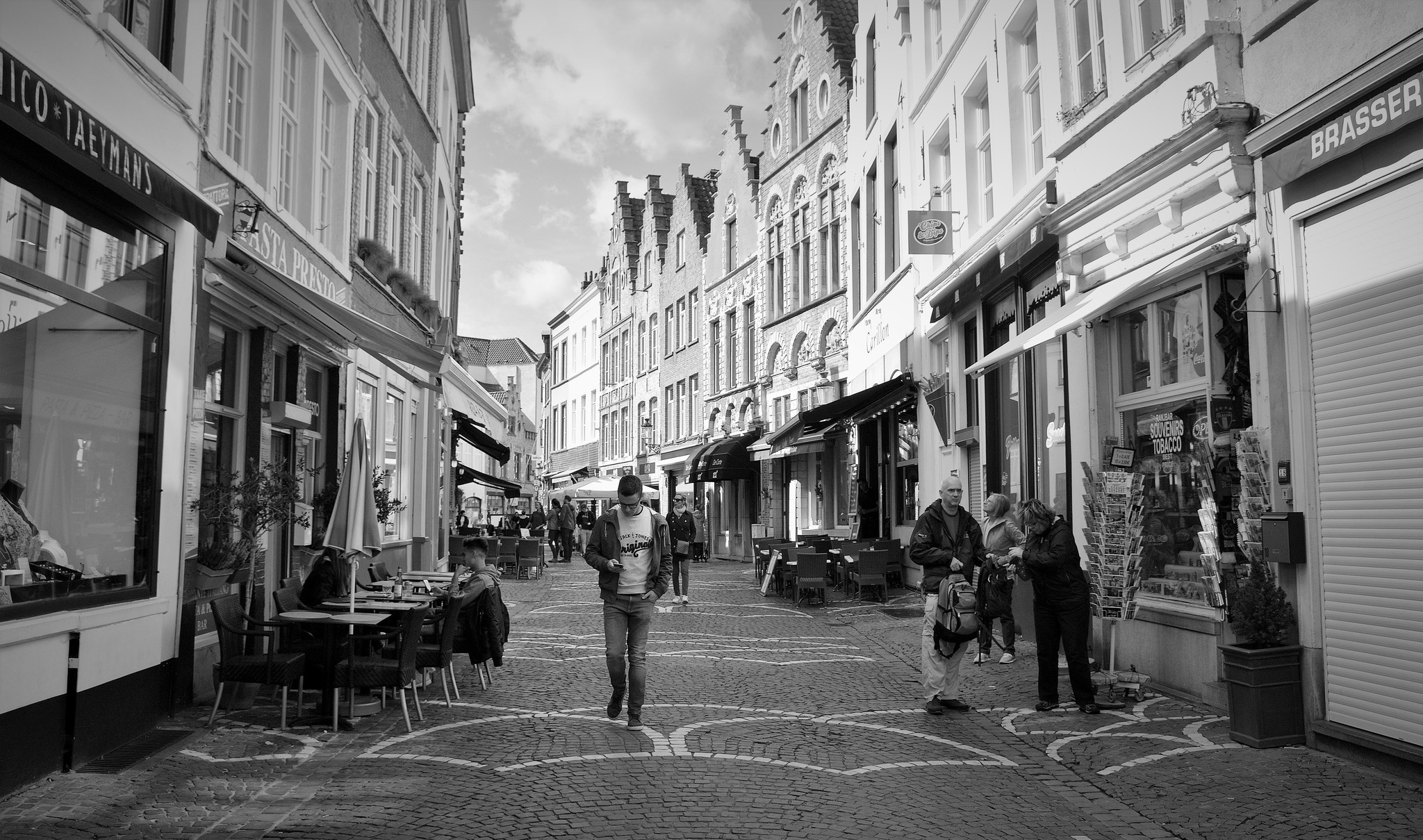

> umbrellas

[{"left": 322, "top": 417, "right": 383, "bottom": 721}]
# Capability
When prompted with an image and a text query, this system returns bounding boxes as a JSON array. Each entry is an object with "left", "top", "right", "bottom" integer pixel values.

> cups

[
  {"left": 382, "top": 584, "right": 392, "bottom": 598},
  {"left": 404, "top": 581, "right": 413, "bottom": 596}
]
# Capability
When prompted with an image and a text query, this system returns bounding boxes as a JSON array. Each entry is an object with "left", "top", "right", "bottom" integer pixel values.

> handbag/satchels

[{"left": 675, "top": 540, "right": 689, "bottom": 556}]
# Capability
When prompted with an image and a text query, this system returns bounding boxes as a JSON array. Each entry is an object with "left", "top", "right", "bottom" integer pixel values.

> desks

[
  {"left": 403, "top": 570, "right": 473, "bottom": 577},
  {"left": 390, "top": 574, "right": 467, "bottom": 582},
  {"left": 350, "top": 591, "right": 438, "bottom": 661},
  {"left": 319, "top": 599, "right": 421, "bottom": 695},
  {"left": 366, "top": 581, "right": 451, "bottom": 648},
  {"left": 269, "top": 609, "right": 391, "bottom": 717}
]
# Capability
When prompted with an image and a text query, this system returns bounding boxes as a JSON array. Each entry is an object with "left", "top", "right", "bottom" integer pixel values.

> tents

[{"left": 549, "top": 474, "right": 659, "bottom": 519}]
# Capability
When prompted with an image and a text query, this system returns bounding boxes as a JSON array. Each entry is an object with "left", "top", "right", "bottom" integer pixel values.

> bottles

[{"left": 394, "top": 566, "right": 403, "bottom": 600}]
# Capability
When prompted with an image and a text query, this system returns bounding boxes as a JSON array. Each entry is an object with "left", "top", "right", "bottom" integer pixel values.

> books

[{"left": 1081, "top": 428, "right": 1270, "bottom": 620}]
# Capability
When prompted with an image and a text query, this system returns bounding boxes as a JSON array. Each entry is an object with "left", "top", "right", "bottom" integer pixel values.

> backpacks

[
  {"left": 975, "top": 555, "right": 1013, "bottom": 620},
  {"left": 932, "top": 567, "right": 978, "bottom": 658}
]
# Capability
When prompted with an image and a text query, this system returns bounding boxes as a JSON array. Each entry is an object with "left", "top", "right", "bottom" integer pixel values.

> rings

[{"left": 40, "top": 565, "right": 67, "bottom": 573}]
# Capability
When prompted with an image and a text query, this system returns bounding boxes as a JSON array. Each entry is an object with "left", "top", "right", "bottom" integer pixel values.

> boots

[{"left": 559, "top": 549, "right": 563, "bottom": 557}]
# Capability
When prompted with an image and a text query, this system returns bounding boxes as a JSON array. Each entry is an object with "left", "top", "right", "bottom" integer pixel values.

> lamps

[
  {"left": 451, "top": 453, "right": 458, "bottom": 467},
  {"left": 459, "top": 465, "right": 465, "bottom": 475},
  {"left": 641, "top": 418, "right": 661, "bottom": 455}
]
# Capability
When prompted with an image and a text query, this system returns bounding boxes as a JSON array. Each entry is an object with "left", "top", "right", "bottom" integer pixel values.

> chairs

[
  {"left": 206, "top": 536, "right": 549, "bottom": 733},
  {"left": 753, "top": 534, "right": 905, "bottom": 608}
]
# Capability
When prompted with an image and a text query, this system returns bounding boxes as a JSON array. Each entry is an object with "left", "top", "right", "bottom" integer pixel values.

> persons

[
  {"left": 404, "top": 538, "right": 503, "bottom": 688},
  {"left": 586, "top": 475, "right": 672, "bottom": 729},
  {"left": 908, "top": 476, "right": 1099, "bottom": 715},
  {"left": 666, "top": 492, "right": 705, "bottom": 604},
  {"left": 299, "top": 548, "right": 382, "bottom": 659},
  {"left": 456, "top": 494, "right": 655, "bottom": 563}
]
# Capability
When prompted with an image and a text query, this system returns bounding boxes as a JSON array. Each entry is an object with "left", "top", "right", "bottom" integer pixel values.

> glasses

[
  {"left": 1024, "top": 521, "right": 1038, "bottom": 527},
  {"left": 672, "top": 498, "right": 682, "bottom": 502},
  {"left": 984, "top": 499, "right": 996, "bottom": 504},
  {"left": 617, "top": 496, "right": 641, "bottom": 508}
]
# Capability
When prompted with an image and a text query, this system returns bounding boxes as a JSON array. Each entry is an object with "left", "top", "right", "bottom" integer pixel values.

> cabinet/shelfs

[
  {"left": 1148, "top": 537, "right": 1204, "bottom": 605},
  {"left": 1083, "top": 473, "right": 1152, "bottom": 702}
]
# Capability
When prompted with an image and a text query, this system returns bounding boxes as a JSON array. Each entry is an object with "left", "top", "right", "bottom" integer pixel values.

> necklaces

[
  {"left": 41, "top": 537, "right": 64, "bottom": 557},
  {"left": 0, "top": 546, "right": 12, "bottom": 564},
  {"left": 0, "top": 491, "right": 21, "bottom": 509}
]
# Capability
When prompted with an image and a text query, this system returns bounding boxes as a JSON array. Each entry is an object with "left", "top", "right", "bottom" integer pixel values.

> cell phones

[
  {"left": 613, "top": 563, "right": 623, "bottom": 568},
  {"left": 423, "top": 580, "right": 434, "bottom": 595}
]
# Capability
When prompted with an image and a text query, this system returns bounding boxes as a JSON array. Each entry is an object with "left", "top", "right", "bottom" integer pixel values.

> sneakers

[
  {"left": 627, "top": 714, "right": 644, "bottom": 730},
  {"left": 1079, "top": 703, "right": 1100, "bottom": 713},
  {"left": 925, "top": 694, "right": 943, "bottom": 714},
  {"left": 940, "top": 698, "right": 969, "bottom": 711},
  {"left": 672, "top": 596, "right": 680, "bottom": 603},
  {"left": 404, "top": 671, "right": 432, "bottom": 689},
  {"left": 607, "top": 688, "right": 627, "bottom": 720},
  {"left": 973, "top": 653, "right": 991, "bottom": 663},
  {"left": 1035, "top": 700, "right": 1059, "bottom": 711},
  {"left": 999, "top": 653, "right": 1016, "bottom": 663},
  {"left": 681, "top": 595, "right": 688, "bottom": 604}
]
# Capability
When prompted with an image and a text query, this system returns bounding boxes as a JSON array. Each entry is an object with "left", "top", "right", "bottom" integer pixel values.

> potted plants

[
  {"left": 1217, "top": 556, "right": 1306, "bottom": 748},
  {"left": 227, "top": 535, "right": 266, "bottom": 582},
  {"left": 196, "top": 533, "right": 239, "bottom": 591}
]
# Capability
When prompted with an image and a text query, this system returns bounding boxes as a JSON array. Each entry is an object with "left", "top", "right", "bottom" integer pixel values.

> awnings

[
  {"left": 684, "top": 230, "right": 1235, "bottom": 483},
  {"left": 215, "top": 232, "right": 521, "bottom": 499}
]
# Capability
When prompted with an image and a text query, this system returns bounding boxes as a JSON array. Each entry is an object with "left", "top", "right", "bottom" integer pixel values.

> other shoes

[
  {"left": 558, "top": 558, "right": 571, "bottom": 563},
  {"left": 548, "top": 558, "right": 558, "bottom": 562}
]
